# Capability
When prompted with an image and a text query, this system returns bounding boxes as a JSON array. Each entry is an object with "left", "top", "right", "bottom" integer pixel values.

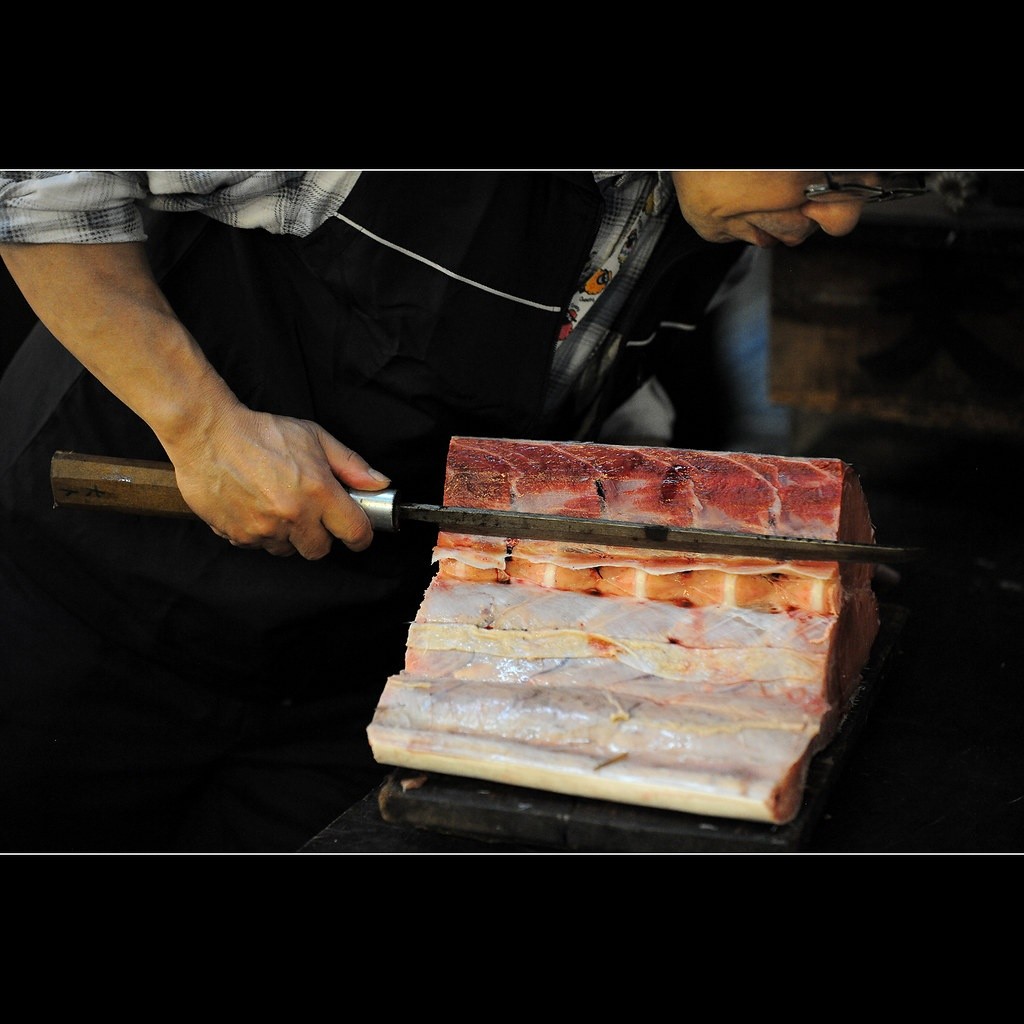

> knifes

[{"left": 47, "top": 445, "right": 917, "bottom": 565}]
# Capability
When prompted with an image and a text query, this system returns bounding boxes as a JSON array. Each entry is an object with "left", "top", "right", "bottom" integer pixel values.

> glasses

[{"left": 806, "top": 172, "right": 928, "bottom": 204}]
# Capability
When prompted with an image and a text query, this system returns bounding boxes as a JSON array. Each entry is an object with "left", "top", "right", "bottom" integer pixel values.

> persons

[{"left": 0, "top": 172, "right": 931, "bottom": 560}]
